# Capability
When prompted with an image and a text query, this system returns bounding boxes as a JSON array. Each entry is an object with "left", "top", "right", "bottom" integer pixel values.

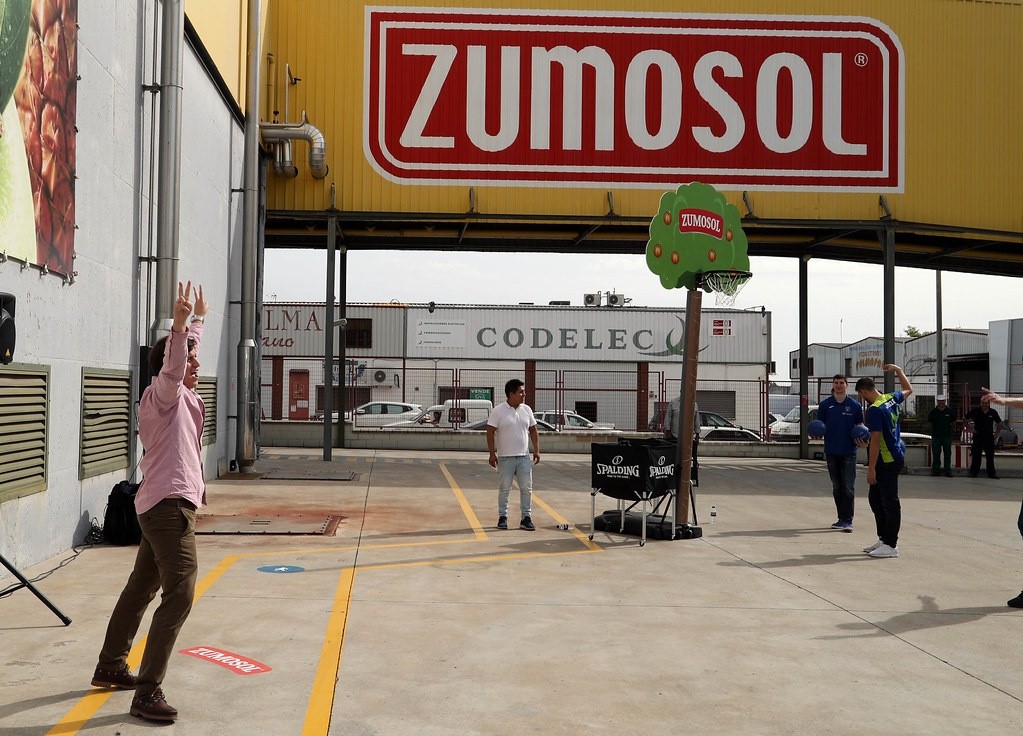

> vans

[
  {"left": 380, "top": 399, "right": 493, "bottom": 431},
  {"left": 768, "top": 403, "right": 824, "bottom": 443}
]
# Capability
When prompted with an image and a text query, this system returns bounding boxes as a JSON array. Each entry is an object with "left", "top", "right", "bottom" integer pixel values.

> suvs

[
  {"left": 648, "top": 408, "right": 760, "bottom": 441},
  {"left": 319, "top": 400, "right": 423, "bottom": 431},
  {"left": 534, "top": 409, "right": 616, "bottom": 430}
]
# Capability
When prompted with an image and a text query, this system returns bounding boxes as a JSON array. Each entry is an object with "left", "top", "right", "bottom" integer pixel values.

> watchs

[{"left": 189, "top": 314, "right": 205, "bottom": 323}]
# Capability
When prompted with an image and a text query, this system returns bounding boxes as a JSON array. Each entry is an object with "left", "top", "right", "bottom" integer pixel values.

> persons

[
  {"left": 928, "top": 394, "right": 953, "bottom": 477},
  {"left": 432, "top": 412, "right": 440, "bottom": 424},
  {"left": 92, "top": 280, "right": 208, "bottom": 721},
  {"left": 665, "top": 388, "right": 701, "bottom": 443},
  {"left": 486, "top": 379, "right": 541, "bottom": 531},
  {"left": 963, "top": 395, "right": 1002, "bottom": 479},
  {"left": 981, "top": 386, "right": 1023, "bottom": 609},
  {"left": 810, "top": 374, "right": 870, "bottom": 532},
  {"left": 854, "top": 364, "right": 914, "bottom": 558}
]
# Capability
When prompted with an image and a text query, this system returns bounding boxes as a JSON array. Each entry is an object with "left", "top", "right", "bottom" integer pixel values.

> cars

[
  {"left": 960, "top": 421, "right": 1019, "bottom": 452},
  {"left": 458, "top": 417, "right": 560, "bottom": 436},
  {"left": 692, "top": 424, "right": 777, "bottom": 442}
]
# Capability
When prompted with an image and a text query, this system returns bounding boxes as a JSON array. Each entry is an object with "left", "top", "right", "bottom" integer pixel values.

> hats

[{"left": 937, "top": 395, "right": 946, "bottom": 401}]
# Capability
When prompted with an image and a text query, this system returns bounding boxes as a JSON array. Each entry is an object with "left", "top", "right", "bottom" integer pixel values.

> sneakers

[
  {"left": 832, "top": 520, "right": 843, "bottom": 528},
  {"left": 130, "top": 692, "right": 178, "bottom": 721},
  {"left": 520, "top": 516, "right": 536, "bottom": 530},
  {"left": 91, "top": 664, "right": 139, "bottom": 690},
  {"left": 842, "top": 521, "right": 853, "bottom": 531},
  {"left": 863, "top": 539, "right": 884, "bottom": 553},
  {"left": 497, "top": 516, "right": 508, "bottom": 528},
  {"left": 869, "top": 543, "right": 899, "bottom": 558}
]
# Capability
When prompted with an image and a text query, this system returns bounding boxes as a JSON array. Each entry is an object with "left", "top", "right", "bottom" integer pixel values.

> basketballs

[
  {"left": 806, "top": 420, "right": 826, "bottom": 436},
  {"left": 850, "top": 425, "right": 870, "bottom": 443}
]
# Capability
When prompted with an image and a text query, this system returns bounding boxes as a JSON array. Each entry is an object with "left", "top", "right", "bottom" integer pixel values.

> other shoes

[
  {"left": 931, "top": 470, "right": 939, "bottom": 475},
  {"left": 945, "top": 472, "right": 954, "bottom": 476},
  {"left": 989, "top": 474, "right": 1000, "bottom": 480},
  {"left": 1007, "top": 590, "right": 1023, "bottom": 608},
  {"left": 968, "top": 473, "right": 977, "bottom": 477}
]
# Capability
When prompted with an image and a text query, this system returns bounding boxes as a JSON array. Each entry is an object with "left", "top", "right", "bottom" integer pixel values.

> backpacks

[{"left": 102, "top": 480, "right": 144, "bottom": 545}]
